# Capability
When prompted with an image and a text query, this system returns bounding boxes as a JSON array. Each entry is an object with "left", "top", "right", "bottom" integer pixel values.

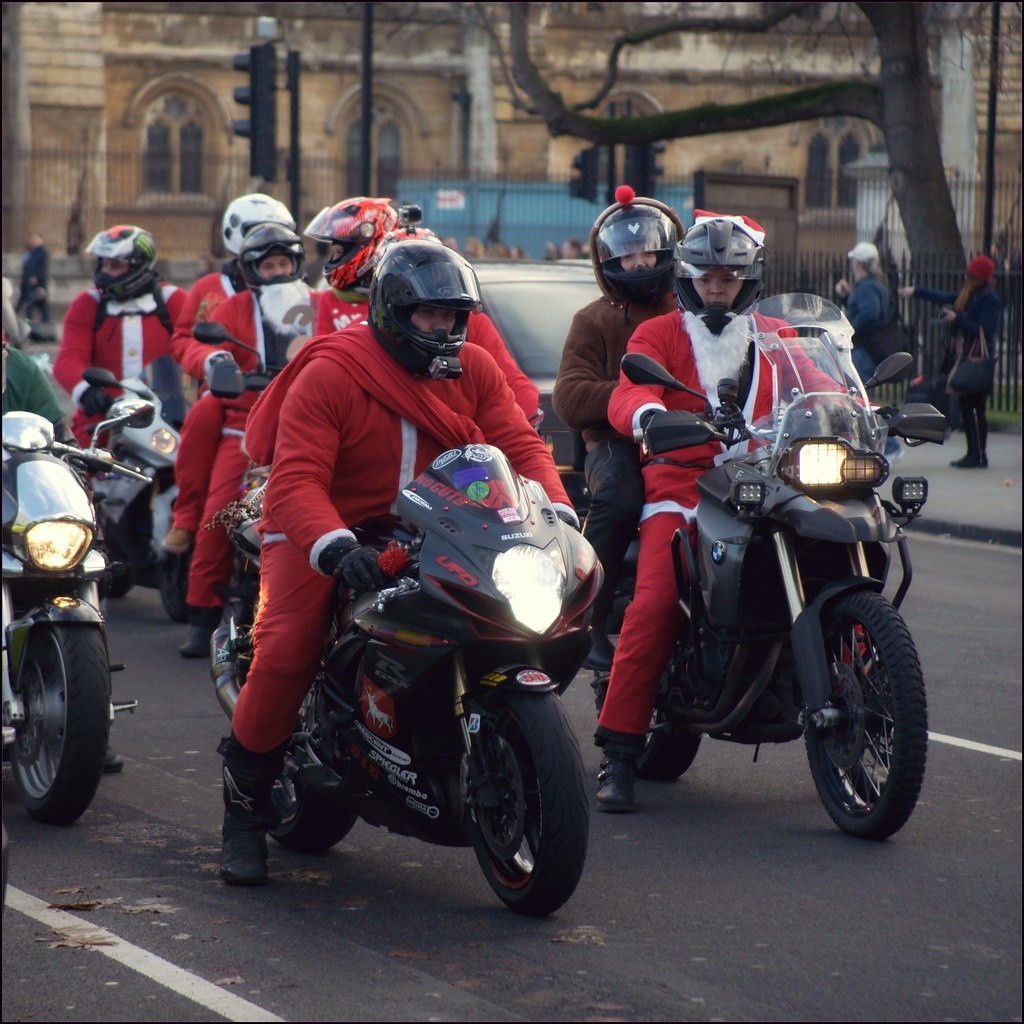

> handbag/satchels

[
  {"left": 863, "top": 317, "right": 915, "bottom": 384},
  {"left": 947, "top": 326, "right": 998, "bottom": 398}
]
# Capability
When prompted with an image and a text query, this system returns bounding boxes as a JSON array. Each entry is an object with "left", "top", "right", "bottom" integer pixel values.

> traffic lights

[
  {"left": 628, "top": 141, "right": 665, "bottom": 199},
  {"left": 232, "top": 44, "right": 275, "bottom": 178},
  {"left": 568, "top": 146, "right": 597, "bottom": 203}
]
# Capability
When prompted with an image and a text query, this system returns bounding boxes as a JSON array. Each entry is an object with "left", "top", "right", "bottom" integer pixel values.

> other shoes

[
  {"left": 182, "top": 625, "right": 217, "bottom": 661},
  {"left": 950, "top": 448, "right": 994, "bottom": 470},
  {"left": 104, "top": 748, "right": 126, "bottom": 772},
  {"left": 584, "top": 629, "right": 618, "bottom": 672},
  {"left": 595, "top": 753, "right": 654, "bottom": 808}
]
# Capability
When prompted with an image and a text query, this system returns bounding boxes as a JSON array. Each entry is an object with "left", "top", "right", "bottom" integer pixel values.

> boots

[{"left": 217, "top": 727, "right": 276, "bottom": 888}]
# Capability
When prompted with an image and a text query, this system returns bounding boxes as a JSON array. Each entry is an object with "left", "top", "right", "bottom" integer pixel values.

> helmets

[
  {"left": 596, "top": 203, "right": 682, "bottom": 302},
  {"left": 84, "top": 224, "right": 165, "bottom": 303},
  {"left": 305, "top": 196, "right": 405, "bottom": 294},
  {"left": 375, "top": 224, "right": 446, "bottom": 275},
  {"left": 365, "top": 240, "right": 487, "bottom": 379},
  {"left": 231, "top": 224, "right": 307, "bottom": 289},
  {"left": 666, "top": 218, "right": 769, "bottom": 336},
  {"left": 219, "top": 192, "right": 301, "bottom": 259}
]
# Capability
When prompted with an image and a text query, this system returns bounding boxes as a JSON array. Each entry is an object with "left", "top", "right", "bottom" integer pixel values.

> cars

[{"left": 467, "top": 260, "right": 604, "bottom": 533}]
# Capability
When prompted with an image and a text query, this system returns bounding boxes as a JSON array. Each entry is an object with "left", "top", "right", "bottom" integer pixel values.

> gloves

[
  {"left": 550, "top": 502, "right": 588, "bottom": 535},
  {"left": 76, "top": 382, "right": 115, "bottom": 417},
  {"left": 305, "top": 528, "right": 399, "bottom": 592},
  {"left": 208, "top": 353, "right": 234, "bottom": 368}
]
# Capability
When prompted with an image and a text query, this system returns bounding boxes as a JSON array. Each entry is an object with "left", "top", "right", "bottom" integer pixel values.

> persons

[
  {"left": 215, "top": 241, "right": 580, "bottom": 883},
  {"left": 836, "top": 242, "right": 905, "bottom": 467},
  {"left": 898, "top": 256, "right": 1000, "bottom": 467},
  {"left": 2, "top": 338, "right": 123, "bottom": 771},
  {"left": 15, "top": 232, "right": 51, "bottom": 323},
  {"left": 440, "top": 236, "right": 590, "bottom": 259},
  {"left": 552, "top": 184, "right": 878, "bottom": 813},
  {"left": 57, "top": 197, "right": 539, "bottom": 656}
]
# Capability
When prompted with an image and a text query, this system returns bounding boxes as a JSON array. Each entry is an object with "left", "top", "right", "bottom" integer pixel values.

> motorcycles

[
  {"left": 83, "top": 355, "right": 192, "bottom": 623},
  {"left": 192, "top": 321, "right": 606, "bottom": 919},
  {"left": 577, "top": 293, "right": 948, "bottom": 841},
  {"left": 2, "top": 399, "right": 155, "bottom": 824}
]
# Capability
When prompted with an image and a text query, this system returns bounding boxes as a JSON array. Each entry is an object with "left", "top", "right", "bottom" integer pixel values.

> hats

[
  {"left": 963, "top": 254, "right": 997, "bottom": 282},
  {"left": 846, "top": 243, "right": 883, "bottom": 262}
]
